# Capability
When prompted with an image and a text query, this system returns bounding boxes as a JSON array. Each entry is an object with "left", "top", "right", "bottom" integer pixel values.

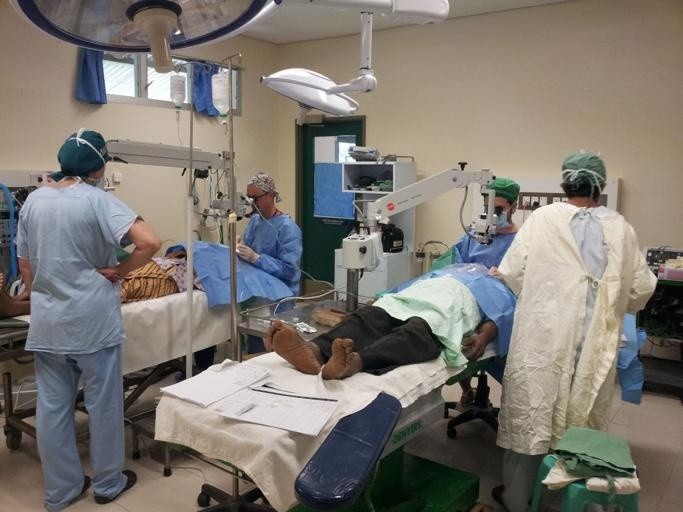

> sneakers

[
  {"left": 94, "top": 469, "right": 136, "bottom": 503},
  {"left": 79, "top": 475, "right": 91, "bottom": 495}
]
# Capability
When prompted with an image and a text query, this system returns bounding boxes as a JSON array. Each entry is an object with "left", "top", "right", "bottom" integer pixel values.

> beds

[
  {"left": 1, "top": 240, "right": 293, "bottom": 450},
  {"left": 154, "top": 262, "right": 514, "bottom": 512}
]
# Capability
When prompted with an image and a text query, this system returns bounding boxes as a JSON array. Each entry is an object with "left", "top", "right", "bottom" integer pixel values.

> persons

[
  {"left": 267, "top": 261, "right": 499, "bottom": 382},
  {"left": 500, "top": 148, "right": 660, "bottom": 512},
  {"left": 12, "top": 126, "right": 165, "bottom": 512},
  {"left": 234, "top": 172, "right": 304, "bottom": 355},
  {"left": 0, "top": 247, "right": 205, "bottom": 320},
  {"left": 449, "top": 177, "right": 524, "bottom": 409}
]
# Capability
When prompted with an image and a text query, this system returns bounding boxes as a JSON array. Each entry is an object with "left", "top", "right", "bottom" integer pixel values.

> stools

[{"left": 528, "top": 454, "right": 639, "bottom": 512}]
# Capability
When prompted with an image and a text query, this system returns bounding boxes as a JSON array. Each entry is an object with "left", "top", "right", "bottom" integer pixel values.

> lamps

[
  {"left": 252, "top": 0, "right": 452, "bottom": 129},
  {"left": 6, "top": 0, "right": 274, "bottom": 78}
]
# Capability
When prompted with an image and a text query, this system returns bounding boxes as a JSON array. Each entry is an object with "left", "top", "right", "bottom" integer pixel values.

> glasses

[{"left": 248, "top": 192, "right": 266, "bottom": 203}]
